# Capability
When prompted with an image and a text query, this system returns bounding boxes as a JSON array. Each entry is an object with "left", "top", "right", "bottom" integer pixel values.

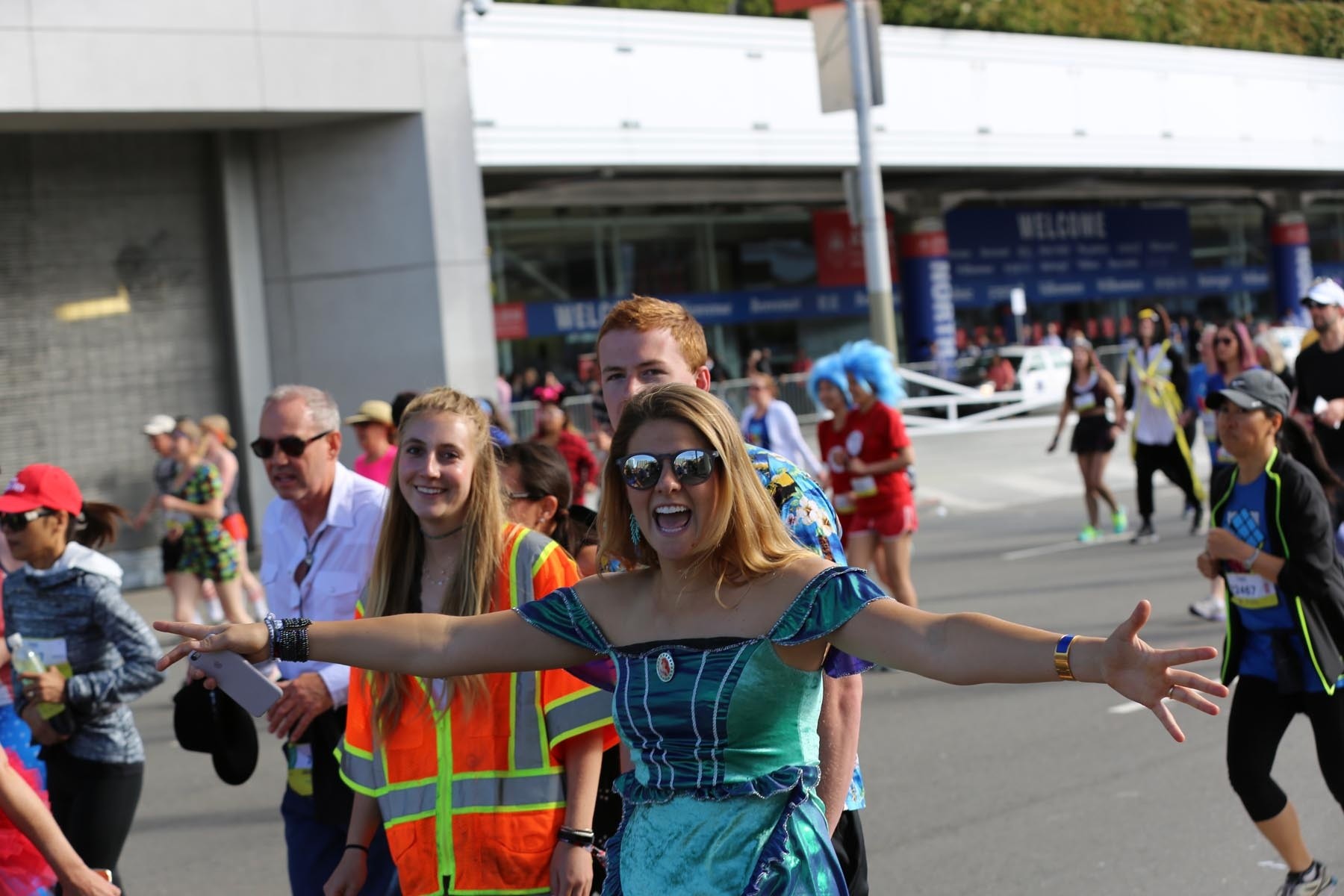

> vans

[{"left": 966, "top": 344, "right": 1075, "bottom": 416}]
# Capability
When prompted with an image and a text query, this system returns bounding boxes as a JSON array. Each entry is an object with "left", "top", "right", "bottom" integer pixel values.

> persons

[{"left": 0, "top": 291, "right": 1344, "bottom": 895}]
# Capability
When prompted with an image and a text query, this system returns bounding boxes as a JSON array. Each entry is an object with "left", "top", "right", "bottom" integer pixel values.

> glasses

[
  {"left": 1308, "top": 301, "right": 1327, "bottom": 309},
  {"left": 250, "top": 428, "right": 334, "bottom": 457},
  {"left": 1212, "top": 337, "right": 1231, "bottom": 345},
  {"left": 615, "top": 447, "right": 720, "bottom": 491},
  {"left": 0, "top": 510, "right": 58, "bottom": 530}
]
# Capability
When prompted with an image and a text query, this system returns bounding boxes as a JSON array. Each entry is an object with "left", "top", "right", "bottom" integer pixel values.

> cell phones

[
  {"left": 1313, "top": 395, "right": 1342, "bottom": 429},
  {"left": 186, "top": 633, "right": 282, "bottom": 719}
]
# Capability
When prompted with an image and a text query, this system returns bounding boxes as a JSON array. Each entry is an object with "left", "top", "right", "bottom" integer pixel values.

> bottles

[{"left": 8, "top": 631, "right": 66, "bottom": 720}]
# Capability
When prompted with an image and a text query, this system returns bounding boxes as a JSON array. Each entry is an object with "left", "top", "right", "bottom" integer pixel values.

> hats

[
  {"left": 1300, "top": 282, "right": 1344, "bottom": 307},
  {"left": 345, "top": 400, "right": 393, "bottom": 427},
  {"left": 143, "top": 414, "right": 176, "bottom": 435},
  {"left": 1205, "top": 368, "right": 1291, "bottom": 422},
  {"left": 0, "top": 465, "right": 82, "bottom": 517},
  {"left": 172, "top": 676, "right": 259, "bottom": 786}
]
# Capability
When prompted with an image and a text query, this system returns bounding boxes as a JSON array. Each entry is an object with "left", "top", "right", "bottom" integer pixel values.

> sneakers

[
  {"left": 1279, "top": 860, "right": 1338, "bottom": 896},
  {"left": 1189, "top": 595, "right": 1226, "bottom": 621},
  {"left": 1190, "top": 508, "right": 1210, "bottom": 535},
  {"left": 1130, "top": 525, "right": 1158, "bottom": 546},
  {"left": 1111, "top": 505, "right": 1127, "bottom": 533},
  {"left": 1077, "top": 526, "right": 1105, "bottom": 542}
]
